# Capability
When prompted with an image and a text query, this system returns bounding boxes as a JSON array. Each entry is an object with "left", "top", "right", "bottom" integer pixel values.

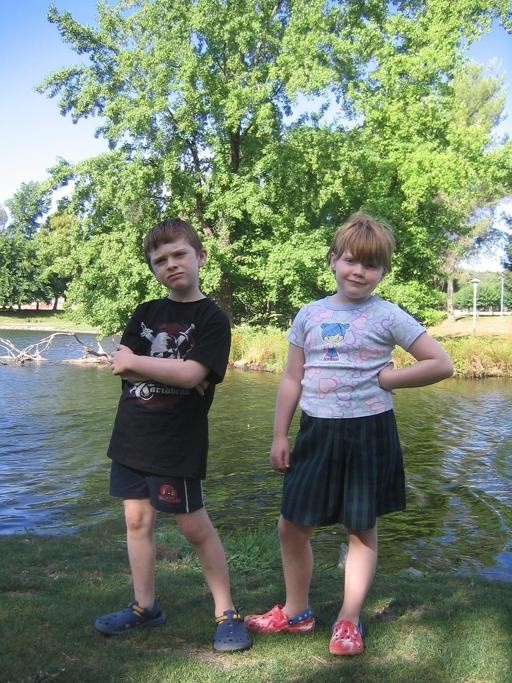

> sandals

[
  {"left": 94, "top": 592, "right": 167, "bottom": 635},
  {"left": 212, "top": 608, "right": 254, "bottom": 654}
]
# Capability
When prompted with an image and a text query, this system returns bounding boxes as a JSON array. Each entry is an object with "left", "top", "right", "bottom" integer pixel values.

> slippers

[
  {"left": 243, "top": 601, "right": 316, "bottom": 636},
  {"left": 327, "top": 612, "right": 367, "bottom": 656}
]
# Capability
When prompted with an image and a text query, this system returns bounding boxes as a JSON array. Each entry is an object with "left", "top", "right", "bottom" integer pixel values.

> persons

[
  {"left": 90, "top": 215, "right": 258, "bottom": 654},
  {"left": 240, "top": 213, "right": 456, "bottom": 660}
]
